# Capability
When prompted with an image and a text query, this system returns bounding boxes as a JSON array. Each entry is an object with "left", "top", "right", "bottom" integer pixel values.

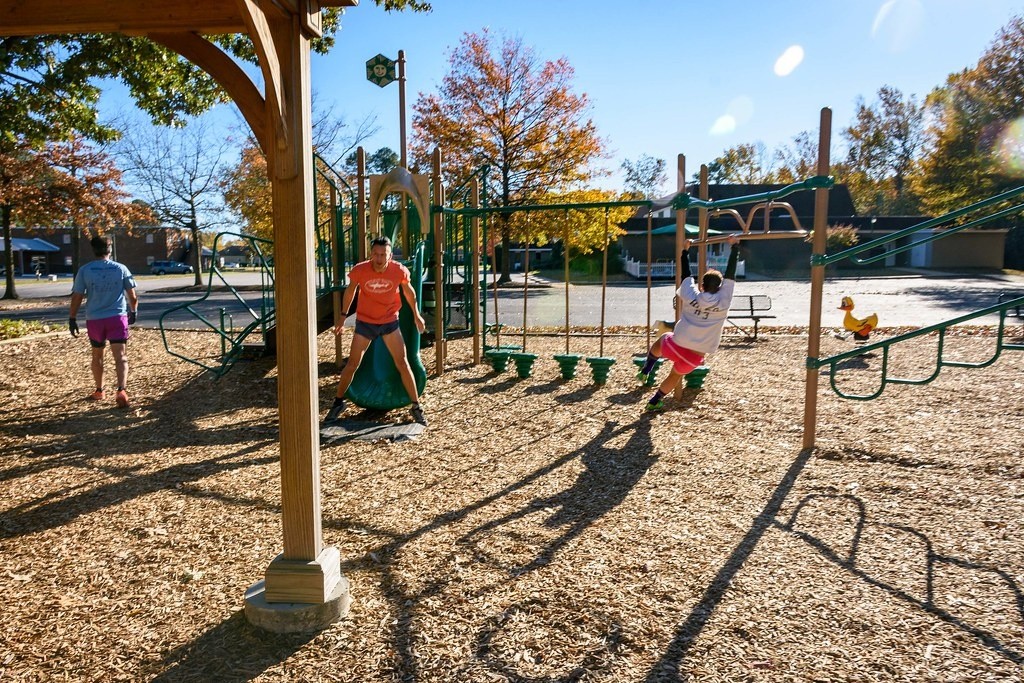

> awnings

[{"left": 0, "top": 236, "right": 60, "bottom": 251}]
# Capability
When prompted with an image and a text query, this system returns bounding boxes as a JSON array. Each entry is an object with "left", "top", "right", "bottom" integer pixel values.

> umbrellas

[{"left": 653, "top": 222, "right": 725, "bottom": 274}]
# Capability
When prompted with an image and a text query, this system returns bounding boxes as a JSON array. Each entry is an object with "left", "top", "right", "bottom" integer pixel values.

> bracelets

[{"left": 341, "top": 312, "right": 347, "bottom": 316}]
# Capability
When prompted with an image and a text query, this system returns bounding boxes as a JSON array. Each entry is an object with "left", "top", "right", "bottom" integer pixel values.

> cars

[
  {"left": 0, "top": 264, "right": 19, "bottom": 276},
  {"left": 223, "top": 261, "right": 240, "bottom": 268}
]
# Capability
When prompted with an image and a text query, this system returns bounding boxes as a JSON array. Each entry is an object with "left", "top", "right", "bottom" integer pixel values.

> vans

[{"left": 152, "top": 260, "right": 194, "bottom": 275}]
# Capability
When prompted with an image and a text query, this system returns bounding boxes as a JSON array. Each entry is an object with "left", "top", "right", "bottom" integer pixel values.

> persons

[
  {"left": 636, "top": 232, "right": 739, "bottom": 410},
  {"left": 69, "top": 236, "right": 139, "bottom": 406},
  {"left": 322, "top": 237, "right": 428, "bottom": 427}
]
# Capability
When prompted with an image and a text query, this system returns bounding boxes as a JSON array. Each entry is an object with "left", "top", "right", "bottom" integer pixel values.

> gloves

[
  {"left": 127, "top": 311, "right": 137, "bottom": 325},
  {"left": 69, "top": 318, "right": 79, "bottom": 338}
]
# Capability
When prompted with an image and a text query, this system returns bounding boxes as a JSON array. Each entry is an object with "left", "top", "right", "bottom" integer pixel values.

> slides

[{"left": 338, "top": 240, "right": 428, "bottom": 413}]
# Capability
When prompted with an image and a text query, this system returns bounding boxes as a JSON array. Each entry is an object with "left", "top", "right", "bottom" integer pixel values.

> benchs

[{"left": 673, "top": 294, "right": 776, "bottom": 340}]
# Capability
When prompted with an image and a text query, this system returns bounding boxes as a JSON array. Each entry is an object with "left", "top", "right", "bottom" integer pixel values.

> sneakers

[
  {"left": 409, "top": 407, "right": 427, "bottom": 427},
  {"left": 323, "top": 401, "right": 347, "bottom": 424},
  {"left": 115, "top": 390, "right": 130, "bottom": 407},
  {"left": 92, "top": 391, "right": 105, "bottom": 400},
  {"left": 644, "top": 401, "right": 664, "bottom": 412},
  {"left": 636, "top": 373, "right": 649, "bottom": 382}
]
